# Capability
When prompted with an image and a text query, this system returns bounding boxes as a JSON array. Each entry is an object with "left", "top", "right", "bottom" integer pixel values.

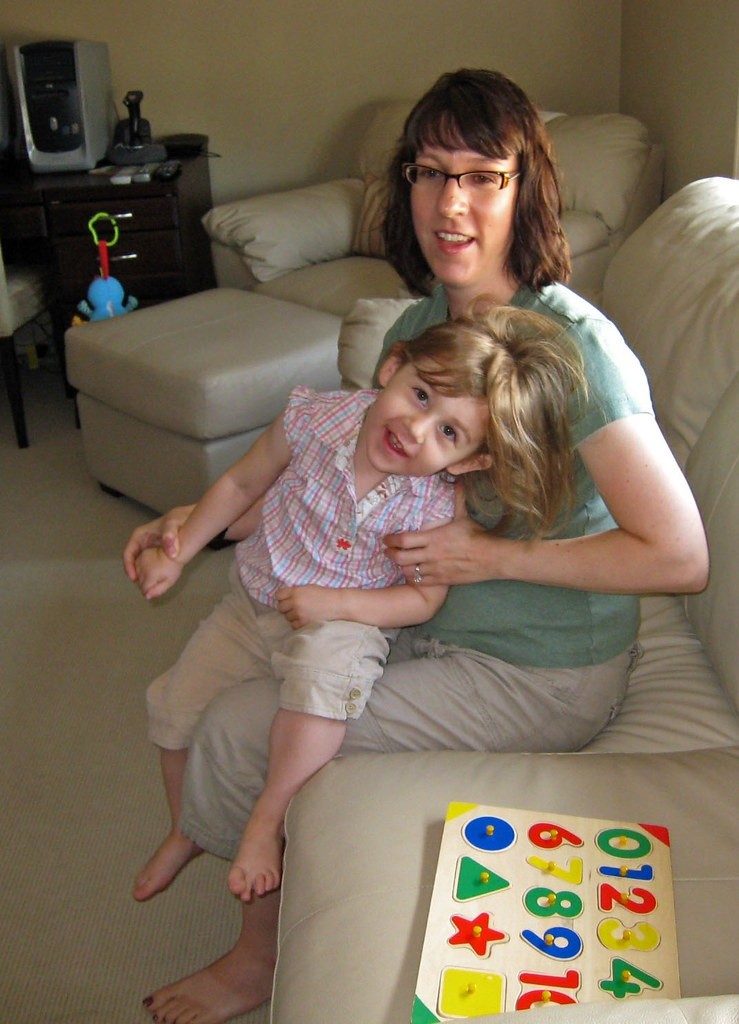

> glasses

[{"left": 401, "top": 163, "right": 521, "bottom": 193}]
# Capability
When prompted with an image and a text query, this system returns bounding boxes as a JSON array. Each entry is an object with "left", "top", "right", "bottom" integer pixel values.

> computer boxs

[{"left": 14, "top": 38, "right": 117, "bottom": 174}]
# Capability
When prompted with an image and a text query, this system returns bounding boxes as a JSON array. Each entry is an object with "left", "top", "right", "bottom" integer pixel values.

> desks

[{"left": 0, "top": 150, "right": 212, "bottom": 319}]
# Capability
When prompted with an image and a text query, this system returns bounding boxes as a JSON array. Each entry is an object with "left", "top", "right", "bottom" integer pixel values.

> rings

[{"left": 414, "top": 563, "right": 423, "bottom": 584}]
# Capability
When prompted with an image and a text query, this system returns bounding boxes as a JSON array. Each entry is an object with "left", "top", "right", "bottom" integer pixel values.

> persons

[
  {"left": 132, "top": 298, "right": 580, "bottom": 904},
  {"left": 119, "top": 68, "right": 711, "bottom": 1023}
]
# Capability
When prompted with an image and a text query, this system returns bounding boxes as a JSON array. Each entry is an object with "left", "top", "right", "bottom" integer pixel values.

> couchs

[
  {"left": 267, "top": 172, "right": 739, "bottom": 1024},
  {"left": 208, "top": 98, "right": 666, "bottom": 294}
]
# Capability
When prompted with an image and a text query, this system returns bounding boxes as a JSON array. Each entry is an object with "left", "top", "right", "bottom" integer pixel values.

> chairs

[{"left": 0, "top": 249, "right": 81, "bottom": 450}]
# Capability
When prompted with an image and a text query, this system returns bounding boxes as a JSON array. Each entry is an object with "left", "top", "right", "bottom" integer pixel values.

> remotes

[{"left": 110, "top": 160, "right": 182, "bottom": 185}]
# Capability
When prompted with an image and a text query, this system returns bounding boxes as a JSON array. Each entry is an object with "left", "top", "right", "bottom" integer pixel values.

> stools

[{"left": 65, "top": 286, "right": 345, "bottom": 552}]
